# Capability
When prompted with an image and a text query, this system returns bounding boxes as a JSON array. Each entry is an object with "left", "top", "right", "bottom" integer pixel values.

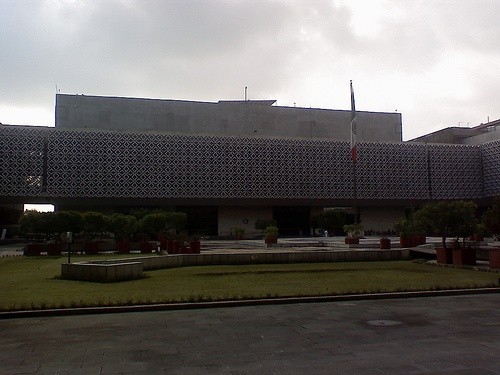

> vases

[{"left": 380, "top": 238, "right": 391, "bottom": 249}]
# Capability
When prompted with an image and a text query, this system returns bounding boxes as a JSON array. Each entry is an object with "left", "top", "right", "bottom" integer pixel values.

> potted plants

[
  {"left": 263, "top": 224, "right": 278, "bottom": 243},
  {"left": 230, "top": 226, "right": 245, "bottom": 240},
  {"left": 343, "top": 223, "right": 362, "bottom": 244},
  {"left": 19, "top": 209, "right": 200, "bottom": 257},
  {"left": 392, "top": 196, "right": 500, "bottom": 268}
]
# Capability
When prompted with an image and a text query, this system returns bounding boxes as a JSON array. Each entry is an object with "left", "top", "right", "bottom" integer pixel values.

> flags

[{"left": 349, "top": 83, "right": 358, "bottom": 160}]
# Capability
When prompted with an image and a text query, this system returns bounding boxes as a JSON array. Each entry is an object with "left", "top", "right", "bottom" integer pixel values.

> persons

[{"left": 325, "top": 230, "right": 328, "bottom": 237}]
